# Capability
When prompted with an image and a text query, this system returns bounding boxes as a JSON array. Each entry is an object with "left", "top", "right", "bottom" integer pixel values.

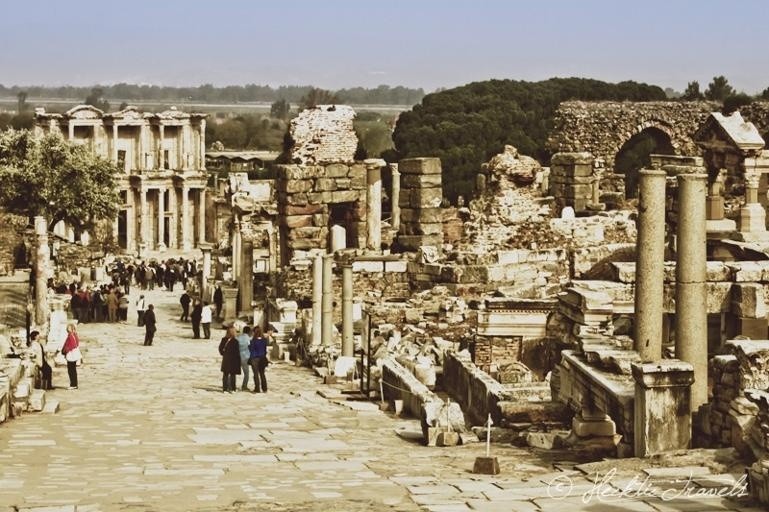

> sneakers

[
  {"left": 67, "top": 386, "right": 78, "bottom": 390},
  {"left": 223, "top": 386, "right": 267, "bottom": 393}
]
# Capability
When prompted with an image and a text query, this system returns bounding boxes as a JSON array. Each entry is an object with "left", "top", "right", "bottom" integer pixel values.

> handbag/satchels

[{"left": 66, "top": 347, "right": 83, "bottom": 362}]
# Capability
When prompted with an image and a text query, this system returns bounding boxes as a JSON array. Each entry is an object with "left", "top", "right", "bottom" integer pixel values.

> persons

[
  {"left": 71, "top": 282, "right": 128, "bottom": 322},
  {"left": 28, "top": 331, "right": 55, "bottom": 389},
  {"left": 180, "top": 289, "right": 212, "bottom": 339},
  {"left": 219, "top": 326, "right": 268, "bottom": 393},
  {"left": 135, "top": 295, "right": 156, "bottom": 346},
  {"left": 111, "top": 257, "right": 198, "bottom": 294},
  {"left": 61, "top": 324, "right": 83, "bottom": 389}
]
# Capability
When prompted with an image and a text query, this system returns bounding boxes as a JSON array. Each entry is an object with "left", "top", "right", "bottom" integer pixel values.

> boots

[{"left": 42, "top": 379, "right": 55, "bottom": 390}]
